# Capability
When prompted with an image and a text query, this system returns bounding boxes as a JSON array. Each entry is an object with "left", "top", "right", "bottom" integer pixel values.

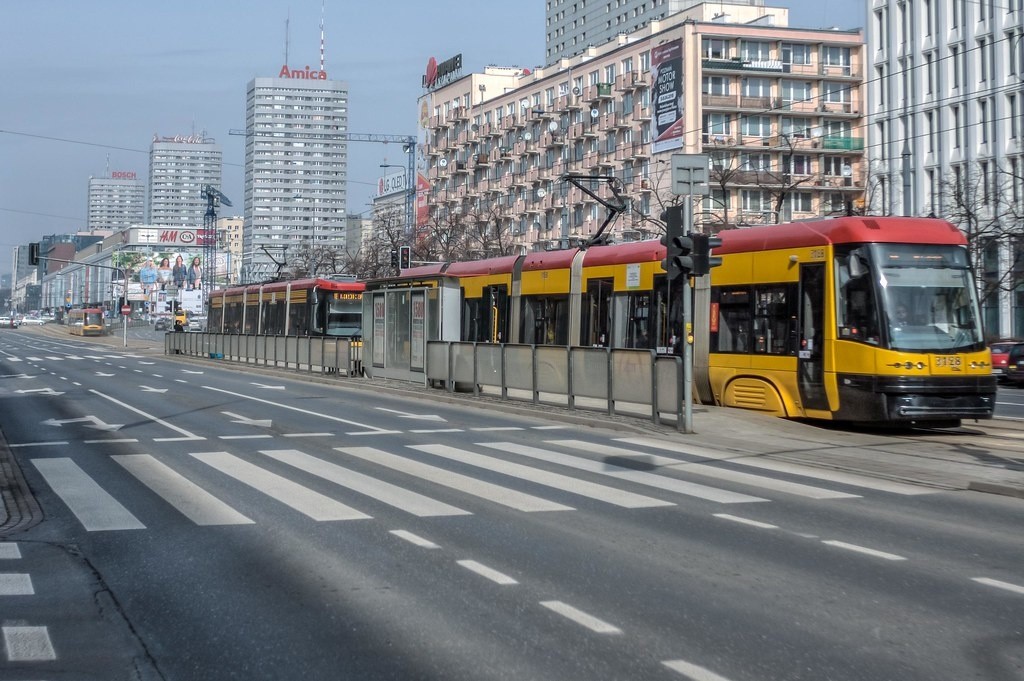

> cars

[
  {"left": 0, "top": 316, "right": 18, "bottom": 329},
  {"left": 21, "top": 316, "right": 46, "bottom": 326}
]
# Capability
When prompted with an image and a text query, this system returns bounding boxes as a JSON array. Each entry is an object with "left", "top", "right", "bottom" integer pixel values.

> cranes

[
  {"left": 199, "top": 182, "right": 233, "bottom": 314},
  {"left": 228, "top": 128, "right": 418, "bottom": 243}
]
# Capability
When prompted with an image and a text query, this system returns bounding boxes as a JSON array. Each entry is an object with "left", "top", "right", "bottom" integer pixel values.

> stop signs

[{"left": 121, "top": 305, "right": 131, "bottom": 315}]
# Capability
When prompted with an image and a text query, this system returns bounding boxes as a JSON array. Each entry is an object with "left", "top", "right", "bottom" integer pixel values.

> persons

[
  {"left": 850, "top": 290, "right": 866, "bottom": 321},
  {"left": 120, "top": 262, "right": 134, "bottom": 278},
  {"left": 139, "top": 257, "right": 157, "bottom": 306},
  {"left": 173, "top": 320, "right": 185, "bottom": 354},
  {"left": 892, "top": 302, "right": 913, "bottom": 329},
  {"left": 635, "top": 328, "right": 649, "bottom": 348},
  {"left": 170, "top": 255, "right": 187, "bottom": 290},
  {"left": 157, "top": 258, "right": 173, "bottom": 291},
  {"left": 10, "top": 316, "right": 13, "bottom": 326},
  {"left": 930, "top": 296, "right": 950, "bottom": 325},
  {"left": 220, "top": 321, "right": 230, "bottom": 334},
  {"left": 186, "top": 257, "right": 204, "bottom": 290},
  {"left": 45, "top": 307, "right": 49, "bottom": 313}
]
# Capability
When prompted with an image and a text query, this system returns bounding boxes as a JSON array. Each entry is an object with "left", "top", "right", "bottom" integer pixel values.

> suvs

[
  {"left": 989, "top": 342, "right": 1023, "bottom": 374},
  {"left": 1006, "top": 343, "right": 1024, "bottom": 381},
  {"left": 155, "top": 321, "right": 166, "bottom": 331}
]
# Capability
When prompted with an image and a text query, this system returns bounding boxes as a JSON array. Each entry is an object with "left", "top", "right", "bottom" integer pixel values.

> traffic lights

[
  {"left": 390, "top": 249, "right": 398, "bottom": 267},
  {"left": 173, "top": 299, "right": 183, "bottom": 313},
  {"left": 673, "top": 232, "right": 698, "bottom": 278},
  {"left": 166, "top": 301, "right": 172, "bottom": 312},
  {"left": 399, "top": 246, "right": 411, "bottom": 271},
  {"left": 659, "top": 206, "right": 680, "bottom": 280},
  {"left": 695, "top": 234, "right": 723, "bottom": 278},
  {"left": 144, "top": 288, "right": 147, "bottom": 295}
]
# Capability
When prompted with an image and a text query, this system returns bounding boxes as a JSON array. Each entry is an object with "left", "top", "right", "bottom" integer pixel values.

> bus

[
  {"left": 364, "top": 173, "right": 997, "bottom": 432},
  {"left": 67, "top": 308, "right": 107, "bottom": 337},
  {"left": 174, "top": 309, "right": 193, "bottom": 326},
  {"left": 206, "top": 244, "right": 366, "bottom": 341}
]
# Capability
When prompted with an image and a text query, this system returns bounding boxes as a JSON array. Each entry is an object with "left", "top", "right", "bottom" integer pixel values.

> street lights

[{"left": 379, "top": 163, "right": 407, "bottom": 239}]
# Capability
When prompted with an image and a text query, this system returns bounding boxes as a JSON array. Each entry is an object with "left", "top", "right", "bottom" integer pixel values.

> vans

[{"left": 189, "top": 318, "right": 203, "bottom": 331}]
